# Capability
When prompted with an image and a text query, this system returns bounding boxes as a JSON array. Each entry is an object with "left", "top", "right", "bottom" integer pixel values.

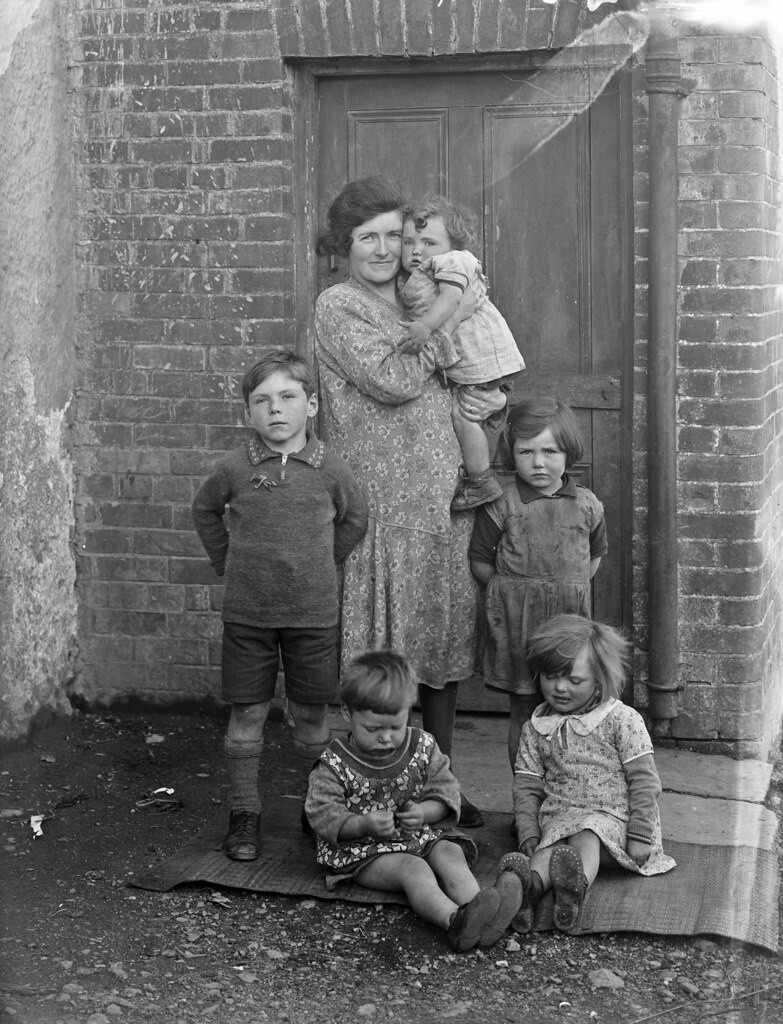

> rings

[{"left": 467, "top": 405, "right": 474, "bottom": 414}]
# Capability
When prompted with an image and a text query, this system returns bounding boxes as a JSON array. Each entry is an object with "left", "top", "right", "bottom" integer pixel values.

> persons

[
  {"left": 299, "top": 649, "right": 525, "bottom": 954},
  {"left": 305, "top": 170, "right": 513, "bottom": 829},
  {"left": 189, "top": 348, "right": 370, "bottom": 862},
  {"left": 465, "top": 396, "right": 611, "bottom": 839},
  {"left": 396, "top": 190, "right": 528, "bottom": 512},
  {"left": 496, "top": 613, "right": 664, "bottom": 935}
]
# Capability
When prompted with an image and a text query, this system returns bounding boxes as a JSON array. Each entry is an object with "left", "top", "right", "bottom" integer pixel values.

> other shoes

[
  {"left": 496, "top": 852, "right": 545, "bottom": 934},
  {"left": 226, "top": 809, "right": 261, "bottom": 860},
  {"left": 450, "top": 467, "right": 504, "bottom": 510},
  {"left": 299, "top": 805, "right": 316, "bottom": 834},
  {"left": 549, "top": 845, "right": 588, "bottom": 931},
  {"left": 446, "top": 888, "right": 501, "bottom": 953},
  {"left": 479, "top": 872, "right": 523, "bottom": 948}
]
